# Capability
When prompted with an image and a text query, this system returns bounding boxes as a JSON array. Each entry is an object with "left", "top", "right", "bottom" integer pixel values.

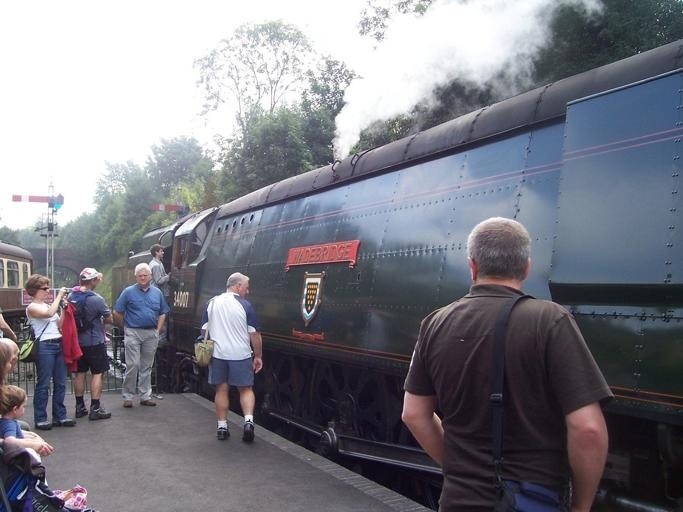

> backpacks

[{"left": 73, "top": 304, "right": 90, "bottom": 333}]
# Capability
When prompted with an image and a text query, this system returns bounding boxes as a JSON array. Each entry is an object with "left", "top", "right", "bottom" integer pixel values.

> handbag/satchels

[
  {"left": 192, "top": 337, "right": 214, "bottom": 368},
  {"left": 491, "top": 478, "right": 571, "bottom": 512},
  {"left": 18, "top": 339, "right": 38, "bottom": 363}
]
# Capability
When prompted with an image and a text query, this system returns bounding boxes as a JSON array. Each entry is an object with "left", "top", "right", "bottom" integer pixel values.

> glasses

[{"left": 36, "top": 286, "right": 51, "bottom": 291}]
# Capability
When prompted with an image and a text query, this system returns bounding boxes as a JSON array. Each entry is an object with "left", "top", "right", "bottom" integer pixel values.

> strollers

[{"left": 0, "top": 441, "right": 97, "bottom": 512}]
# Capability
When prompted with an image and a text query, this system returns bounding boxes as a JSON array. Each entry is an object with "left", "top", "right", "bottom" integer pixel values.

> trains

[
  {"left": 0, "top": 240, "right": 33, "bottom": 332},
  {"left": 105, "top": 39, "right": 682, "bottom": 511}
]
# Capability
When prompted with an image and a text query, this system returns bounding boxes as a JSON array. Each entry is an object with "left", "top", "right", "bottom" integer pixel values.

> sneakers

[
  {"left": 75, "top": 403, "right": 88, "bottom": 419},
  {"left": 122, "top": 400, "right": 133, "bottom": 408},
  {"left": 52, "top": 417, "right": 77, "bottom": 428},
  {"left": 242, "top": 423, "right": 255, "bottom": 443},
  {"left": 216, "top": 425, "right": 231, "bottom": 440},
  {"left": 35, "top": 420, "right": 53, "bottom": 431},
  {"left": 89, "top": 406, "right": 112, "bottom": 420},
  {"left": 140, "top": 399, "right": 156, "bottom": 407}
]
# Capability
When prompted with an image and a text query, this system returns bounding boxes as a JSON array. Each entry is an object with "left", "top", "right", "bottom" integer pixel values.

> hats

[{"left": 79, "top": 267, "right": 101, "bottom": 281}]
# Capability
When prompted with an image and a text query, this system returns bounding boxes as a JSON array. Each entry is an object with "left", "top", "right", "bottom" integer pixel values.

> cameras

[{"left": 60, "top": 288, "right": 77, "bottom": 309}]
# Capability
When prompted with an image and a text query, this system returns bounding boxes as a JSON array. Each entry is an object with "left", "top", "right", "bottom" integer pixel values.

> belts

[{"left": 42, "top": 338, "right": 63, "bottom": 343}]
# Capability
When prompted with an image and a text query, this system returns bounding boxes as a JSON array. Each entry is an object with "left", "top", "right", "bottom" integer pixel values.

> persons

[
  {"left": 0, "top": 336, "right": 23, "bottom": 373},
  {"left": 0, "top": 385, "right": 43, "bottom": 451},
  {"left": 197, "top": 272, "right": 264, "bottom": 443},
  {"left": 114, "top": 262, "right": 169, "bottom": 407},
  {"left": 24, "top": 274, "right": 75, "bottom": 429},
  {"left": 398, "top": 215, "right": 612, "bottom": 510},
  {"left": 0, "top": 310, "right": 17, "bottom": 340},
  {"left": 0, "top": 341, "right": 53, "bottom": 456},
  {"left": 148, "top": 244, "right": 170, "bottom": 288},
  {"left": 64, "top": 269, "right": 112, "bottom": 419}
]
